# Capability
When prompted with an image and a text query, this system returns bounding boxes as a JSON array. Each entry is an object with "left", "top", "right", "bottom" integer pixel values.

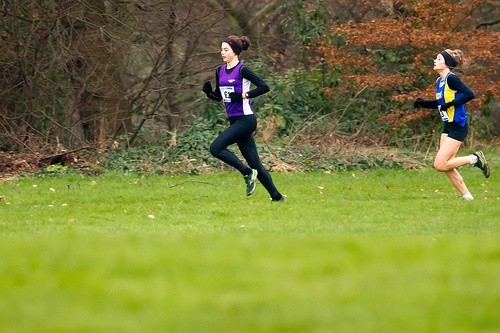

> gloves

[
  {"left": 229, "top": 92, "right": 243, "bottom": 102},
  {"left": 202, "top": 81, "right": 213, "bottom": 96},
  {"left": 439, "top": 101, "right": 455, "bottom": 111},
  {"left": 414, "top": 97, "right": 423, "bottom": 109}
]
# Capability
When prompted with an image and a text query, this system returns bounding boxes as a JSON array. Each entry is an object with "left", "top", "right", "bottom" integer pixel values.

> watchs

[{"left": 245, "top": 92, "right": 249, "bottom": 99}]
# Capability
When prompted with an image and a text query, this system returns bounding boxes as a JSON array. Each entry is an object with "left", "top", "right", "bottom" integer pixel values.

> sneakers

[
  {"left": 244, "top": 169, "right": 257, "bottom": 196},
  {"left": 473, "top": 150, "right": 491, "bottom": 178},
  {"left": 269, "top": 194, "right": 287, "bottom": 202}
]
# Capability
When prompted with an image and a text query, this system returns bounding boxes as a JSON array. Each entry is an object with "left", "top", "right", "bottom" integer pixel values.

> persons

[
  {"left": 201, "top": 35, "right": 284, "bottom": 202},
  {"left": 414, "top": 48, "right": 490, "bottom": 200}
]
